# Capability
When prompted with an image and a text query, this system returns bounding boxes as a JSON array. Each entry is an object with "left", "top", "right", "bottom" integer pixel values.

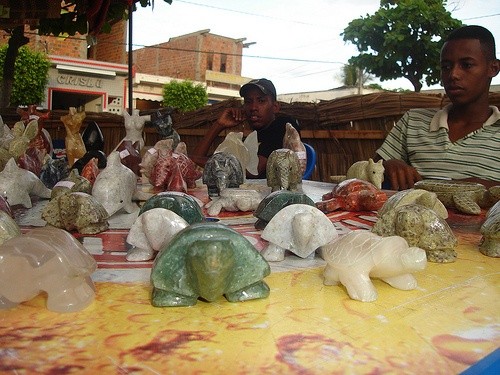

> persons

[
  {"left": 372, "top": 25, "right": 500, "bottom": 210},
  {"left": 190, "top": 78, "right": 301, "bottom": 181}
]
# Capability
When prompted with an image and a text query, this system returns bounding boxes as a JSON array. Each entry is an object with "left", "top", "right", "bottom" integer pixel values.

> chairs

[{"left": 302, "top": 142, "right": 317, "bottom": 180}]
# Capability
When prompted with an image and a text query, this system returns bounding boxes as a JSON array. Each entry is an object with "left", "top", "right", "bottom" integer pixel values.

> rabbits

[{"left": 9, "top": 120, "right": 51, "bottom": 169}]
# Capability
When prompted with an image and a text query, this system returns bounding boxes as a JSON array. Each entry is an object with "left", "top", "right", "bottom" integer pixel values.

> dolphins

[
  {"left": 1, "top": 158, "right": 52, "bottom": 209},
  {"left": 91, "top": 151, "right": 138, "bottom": 221}
]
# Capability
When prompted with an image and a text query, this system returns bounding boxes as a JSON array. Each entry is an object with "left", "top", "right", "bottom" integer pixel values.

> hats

[{"left": 240, "top": 78, "right": 277, "bottom": 100}]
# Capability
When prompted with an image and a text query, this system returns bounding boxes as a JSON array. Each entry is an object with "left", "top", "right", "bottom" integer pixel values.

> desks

[{"left": 0, "top": 178, "right": 500, "bottom": 375}]
